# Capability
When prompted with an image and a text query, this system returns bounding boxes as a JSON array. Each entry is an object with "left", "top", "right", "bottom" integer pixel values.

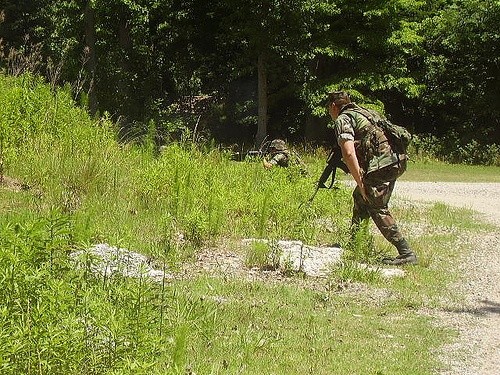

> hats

[
  {"left": 269, "top": 139, "right": 288, "bottom": 150},
  {"left": 322, "top": 90, "right": 349, "bottom": 107}
]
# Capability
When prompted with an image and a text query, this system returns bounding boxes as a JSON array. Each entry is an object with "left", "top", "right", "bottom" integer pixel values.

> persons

[
  {"left": 326, "top": 91, "right": 417, "bottom": 266},
  {"left": 259, "top": 139, "right": 307, "bottom": 179}
]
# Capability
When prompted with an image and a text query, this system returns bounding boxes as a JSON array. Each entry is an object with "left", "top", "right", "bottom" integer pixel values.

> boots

[{"left": 382, "top": 238, "right": 416, "bottom": 268}]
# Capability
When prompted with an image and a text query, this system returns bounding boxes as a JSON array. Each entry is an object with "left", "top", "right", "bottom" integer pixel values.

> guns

[
  {"left": 236, "top": 150, "right": 269, "bottom": 157},
  {"left": 310, "top": 143, "right": 350, "bottom": 202}
]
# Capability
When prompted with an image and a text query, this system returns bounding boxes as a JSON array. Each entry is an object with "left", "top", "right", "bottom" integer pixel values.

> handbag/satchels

[{"left": 382, "top": 121, "right": 411, "bottom": 153}]
